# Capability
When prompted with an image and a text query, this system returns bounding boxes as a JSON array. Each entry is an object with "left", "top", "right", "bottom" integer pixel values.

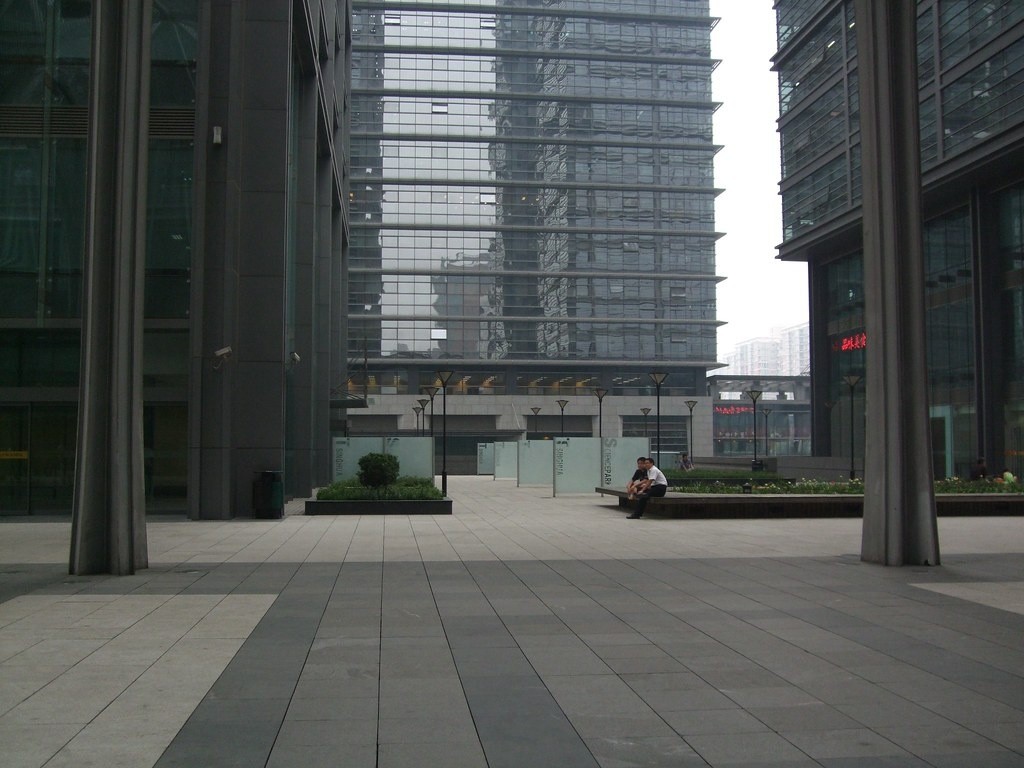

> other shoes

[{"left": 626, "top": 514, "right": 640, "bottom": 518}]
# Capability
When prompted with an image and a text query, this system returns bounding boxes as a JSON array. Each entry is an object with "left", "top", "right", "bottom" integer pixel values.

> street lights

[
  {"left": 530, "top": 407, "right": 542, "bottom": 439},
  {"left": 431, "top": 371, "right": 457, "bottom": 496},
  {"left": 648, "top": 372, "right": 669, "bottom": 469},
  {"left": 683, "top": 400, "right": 698, "bottom": 465},
  {"left": 417, "top": 399, "right": 430, "bottom": 435},
  {"left": 555, "top": 399, "right": 570, "bottom": 438},
  {"left": 423, "top": 387, "right": 441, "bottom": 434},
  {"left": 592, "top": 388, "right": 608, "bottom": 438},
  {"left": 759, "top": 408, "right": 773, "bottom": 457},
  {"left": 639, "top": 407, "right": 652, "bottom": 436},
  {"left": 744, "top": 390, "right": 764, "bottom": 461},
  {"left": 412, "top": 406, "right": 425, "bottom": 436},
  {"left": 842, "top": 373, "right": 865, "bottom": 479}
]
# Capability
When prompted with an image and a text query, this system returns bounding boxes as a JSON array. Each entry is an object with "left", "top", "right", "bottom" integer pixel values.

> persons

[
  {"left": 969, "top": 457, "right": 1018, "bottom": 483},
  {"left": 625, "top": 457, "right": 668, "bottom": 519},
  {"left": 680, "top": 454, "right": 694, "bottom": 471},
  {"left": 626, "top": 457, "right": 651, "bottom": 501}
]
